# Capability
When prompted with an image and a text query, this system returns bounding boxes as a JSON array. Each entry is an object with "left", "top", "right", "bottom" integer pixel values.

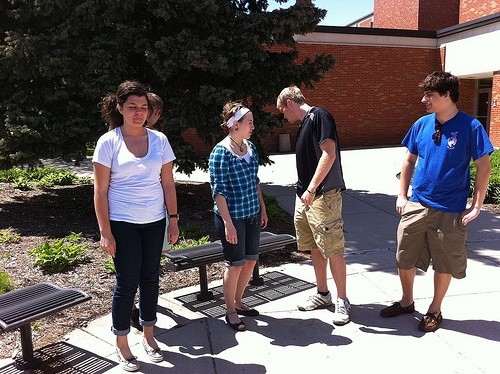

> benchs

[
  {"left": 0, "top": 280, "right": 97, "bottom": 370},
  {"left": 161, "top": 230, "right": 299, "bottom": 302}
]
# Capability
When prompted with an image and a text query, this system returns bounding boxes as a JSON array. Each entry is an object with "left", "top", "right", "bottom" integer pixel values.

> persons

[
  {"left": 276, "top": 87, "right": 351, "bottom": 325},
  {"left": 93, "top": 81, "right": 180, "bottom": 372},
  {"left": 209, "top": 102, "right": 268, "bottom": 330},
  {"left": 380, "top": 70, "right": 495, "bottom": 331}
]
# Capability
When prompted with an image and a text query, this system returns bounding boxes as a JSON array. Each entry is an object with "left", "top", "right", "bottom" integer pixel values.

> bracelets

[{"left": 306, "top": 188, "right": 316, "bottom": 194}]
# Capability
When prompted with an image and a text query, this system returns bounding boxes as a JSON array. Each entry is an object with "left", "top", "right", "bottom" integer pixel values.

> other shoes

[
  {"left": 418, "top": 309, "right": 443, "bottom": 332},
  {"left": 140, "top": 337, "right": 164, "bottom": 363},
  {"left": 116, "top": 347, "right": 139, "bottom": 372},
  {"left": 130, "top": 308, "right": 141, "bottom": 328},
  {"left": 225, "top": 312, "right": 246, "bottom": 331},
  {"left": 235, "top": 303, "right": 259, "bottom": 316},
  {"left": 380, "top": 301, "right": 415, "bottom": 318}
]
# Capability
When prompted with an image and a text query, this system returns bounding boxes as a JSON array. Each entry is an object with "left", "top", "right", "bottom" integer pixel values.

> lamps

[{"left": 493, "top": 99, "right": 497, "bottom": 108}]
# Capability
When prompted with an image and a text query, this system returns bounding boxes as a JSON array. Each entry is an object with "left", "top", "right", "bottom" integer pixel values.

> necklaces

[{"left": 227, "top": 134, "right": 244, "bottom": 152}]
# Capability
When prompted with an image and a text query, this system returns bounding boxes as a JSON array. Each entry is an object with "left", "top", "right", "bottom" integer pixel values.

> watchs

[{"left": 168, "top": 214, "right": 179, "bottom": 220}]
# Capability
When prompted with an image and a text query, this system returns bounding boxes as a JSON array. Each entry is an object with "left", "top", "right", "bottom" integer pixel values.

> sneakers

[
  {"left": 333, "top": 297, "right": 351, "bottom": 325},
  {"left": 297, "top": 291, "right": 332, "bottom": 311}
]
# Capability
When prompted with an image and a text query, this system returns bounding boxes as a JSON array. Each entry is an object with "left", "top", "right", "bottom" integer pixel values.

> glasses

[{"left": 432, "top": 124, "right": 441, "bottom": 142}]
